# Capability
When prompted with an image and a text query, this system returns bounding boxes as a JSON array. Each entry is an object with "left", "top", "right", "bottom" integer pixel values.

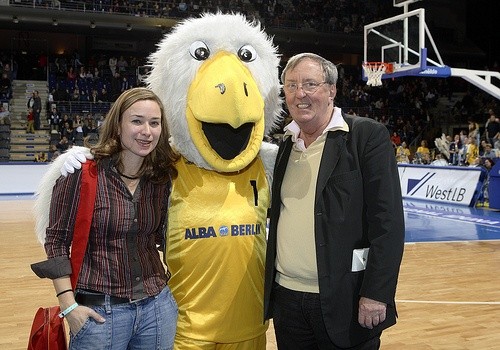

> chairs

[
  {"left": 0, "top": 125, "right": 10, "bottom": 160},
  {"left": 50, "top": 132, "right": 103, "bottom": 161}
]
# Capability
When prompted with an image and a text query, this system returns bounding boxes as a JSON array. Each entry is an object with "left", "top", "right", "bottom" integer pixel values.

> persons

[
  {"left": 0, "top": 0, "right": 500, "bottom": 207},
  {"left": 264, "top": 53, "right": 405, "bottom": 350},
  {"left": 30, "top": 87, "right": 182, "bottom": 350}
]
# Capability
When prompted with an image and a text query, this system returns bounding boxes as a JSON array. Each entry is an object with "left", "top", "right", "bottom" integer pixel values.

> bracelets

[
  {"left": 56, "top": 289, "right": 73, "bottom": 297},
  {"left": 59, "top": 303, "right": 79, "bottom": 318}
]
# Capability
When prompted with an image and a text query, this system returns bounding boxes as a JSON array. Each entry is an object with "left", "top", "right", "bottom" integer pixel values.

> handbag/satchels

[{"left": 28, "top": 306, "right": 67, "bottom": 350}]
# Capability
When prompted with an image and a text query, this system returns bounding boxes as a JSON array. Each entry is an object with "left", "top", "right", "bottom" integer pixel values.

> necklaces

[{"left": 115, "top": 167, "right": 139, "bottom": 180}]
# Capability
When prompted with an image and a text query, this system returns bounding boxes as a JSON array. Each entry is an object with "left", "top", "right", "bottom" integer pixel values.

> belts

[{"left": 75, "top": 293, "right": 148, "bottom": 306}]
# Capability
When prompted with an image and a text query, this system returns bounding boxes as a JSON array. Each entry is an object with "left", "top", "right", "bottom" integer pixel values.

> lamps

[
  {"left": 53, "top": 18, "right": 59, "bottom": 26},
  {"left": 90, "top": 21, "right": 97, "bottom": 29},
  {"left": 126, "top": 24, "right": 132, "bottom": 31},
  {"left": 13, "top": 16, "right": 19, "bottom": 23}
]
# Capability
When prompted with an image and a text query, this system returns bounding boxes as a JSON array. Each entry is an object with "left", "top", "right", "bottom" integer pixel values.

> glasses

[{"left": 283, "top": 82, "right": 332, "bottom": 93}]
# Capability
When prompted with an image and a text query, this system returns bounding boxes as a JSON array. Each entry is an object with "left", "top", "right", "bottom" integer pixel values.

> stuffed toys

[{"left": 35, "top": 12, "right": 281, "bottom": 350}]
